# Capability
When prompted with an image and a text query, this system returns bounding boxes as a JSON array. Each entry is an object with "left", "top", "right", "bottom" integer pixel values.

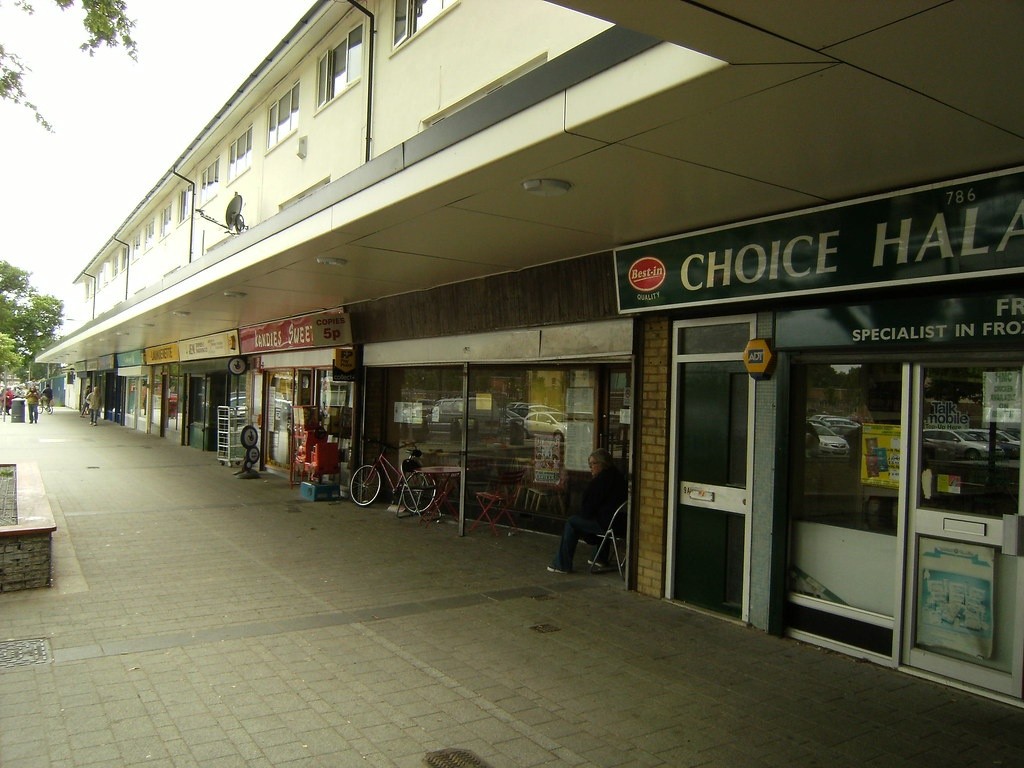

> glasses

[{"left": 590, "top": 461, "right": 599, "bottom": 465}]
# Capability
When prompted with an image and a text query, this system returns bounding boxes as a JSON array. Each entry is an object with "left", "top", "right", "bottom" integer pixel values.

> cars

[
  {"left": 228, "top": 396, "right": 290, "bottom": 430},
  {"left": 806, "top": 415, "right": 1020, "bottom": 465},
  {"left": 416, "top": 397, "right": 569, "bottom": 448}
]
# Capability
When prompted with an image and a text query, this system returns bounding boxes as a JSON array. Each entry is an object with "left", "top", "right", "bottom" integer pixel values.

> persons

[
  {"left": 79, "top": 385, "right": 91, "bottom": 418},
  {"left": 26, "top": 385, "right": 41, "bottom": 424},
  {"left": 86, "top": 387, "right": 102, "bottom": 426},
  {"left": 547, "top": 447, "right": 629, "bottom": 574},
  {"left": 0, "top": 380, "right": 14, "bottom": 415},
  {"left": 39, "top": 384, "right": 53, "bottom": 408}
]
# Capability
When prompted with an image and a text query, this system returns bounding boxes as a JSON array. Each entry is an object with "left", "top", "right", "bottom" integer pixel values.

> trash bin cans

[{"left": 11, "top": 398, "right": 26, "bottom": 423}]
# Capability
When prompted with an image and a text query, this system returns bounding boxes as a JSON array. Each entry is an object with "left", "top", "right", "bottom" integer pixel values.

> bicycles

[
  {"left": 37, "top": 401, "right": 54, "bottom": 415},
  {"left": 349, "top": 439, "right": 437, "bottom": 514}
]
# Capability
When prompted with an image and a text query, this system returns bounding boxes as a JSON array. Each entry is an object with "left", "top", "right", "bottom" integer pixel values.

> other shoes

[
  {"left": 94, "top": 422, "right": 97, "bottom": 426},
  {"left": 547, "top": 565, "right": 568, "bottom": 574},
  {"left": 35, "top": 420, "right": 37, "bottom": 423},
  {"left": 80, "top": 414, "right": 85, "bottom": 418},
  {"left": 588, "top": 559, "right": 606, "bottom": 568},
  {"left": 29, "top": 420, "right": 33, "bottom": 423},
  {"left": 90, "top": 422, "right": 92, "bottom": 425}
]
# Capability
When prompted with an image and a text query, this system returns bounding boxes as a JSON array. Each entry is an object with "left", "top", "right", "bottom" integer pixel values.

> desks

[{"left": 417, "top": 466, "right": 463, "bottom": 528}]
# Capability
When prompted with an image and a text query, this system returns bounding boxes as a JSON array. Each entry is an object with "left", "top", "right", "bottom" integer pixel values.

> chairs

[
  {"left": 468, "top": 468, "right": 526, "bottom": 535},
  {"left": 589, "top": 500, "right": 629, "bottom": 579}
]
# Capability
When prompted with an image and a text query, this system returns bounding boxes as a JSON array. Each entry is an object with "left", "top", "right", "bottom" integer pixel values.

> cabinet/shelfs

[{"left": 218, "top": 405, "right": 248, "bottom": 462}]
